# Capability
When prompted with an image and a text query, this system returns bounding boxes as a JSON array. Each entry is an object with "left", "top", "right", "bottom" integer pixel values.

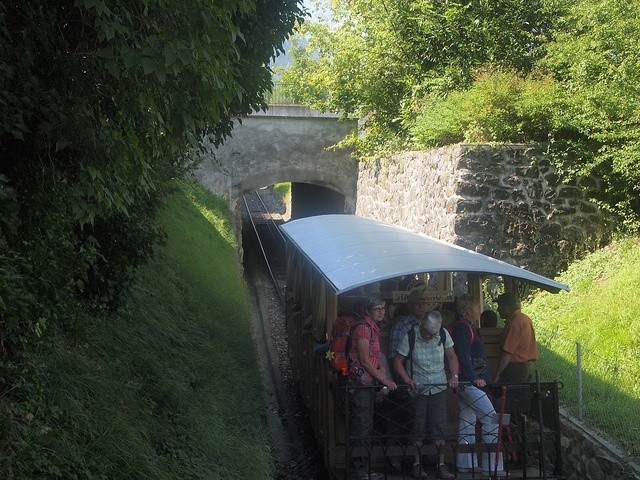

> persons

[
  {"left": 387, "top": 290, "right": 429, "bottom": 368},
  {"left": 479, "top": 309, "right": 498, "bottom": 327},
  {"left": 448, "top": 294, "right": 513, "bottom": 478},
  {"left": 344, "top": 292, "right": 398, "bottom": 478},
  {"left": 493, "top": 291, "right": 539, "bottom": 471},
  {"left": 393, "top": 311, "right": 460, "bottom": 479}
]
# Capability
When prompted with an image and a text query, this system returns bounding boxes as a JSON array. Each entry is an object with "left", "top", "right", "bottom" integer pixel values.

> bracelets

[{"left": 451, "top": 374, "right": 458, "bottom": 378}]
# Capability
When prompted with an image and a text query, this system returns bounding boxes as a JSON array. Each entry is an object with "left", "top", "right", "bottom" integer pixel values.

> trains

[{"left": 278, "top": 214, "right": 570, "bottom": 480}]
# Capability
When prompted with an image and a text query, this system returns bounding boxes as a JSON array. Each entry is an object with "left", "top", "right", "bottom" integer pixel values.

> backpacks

[{"left": 327, "top": 320, "right": 354, "bottom": 377}]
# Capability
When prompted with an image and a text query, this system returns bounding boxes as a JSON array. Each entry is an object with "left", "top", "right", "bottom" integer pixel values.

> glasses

[{"left": 370, "top": 306, "right": 387, "bottom": 312}]
